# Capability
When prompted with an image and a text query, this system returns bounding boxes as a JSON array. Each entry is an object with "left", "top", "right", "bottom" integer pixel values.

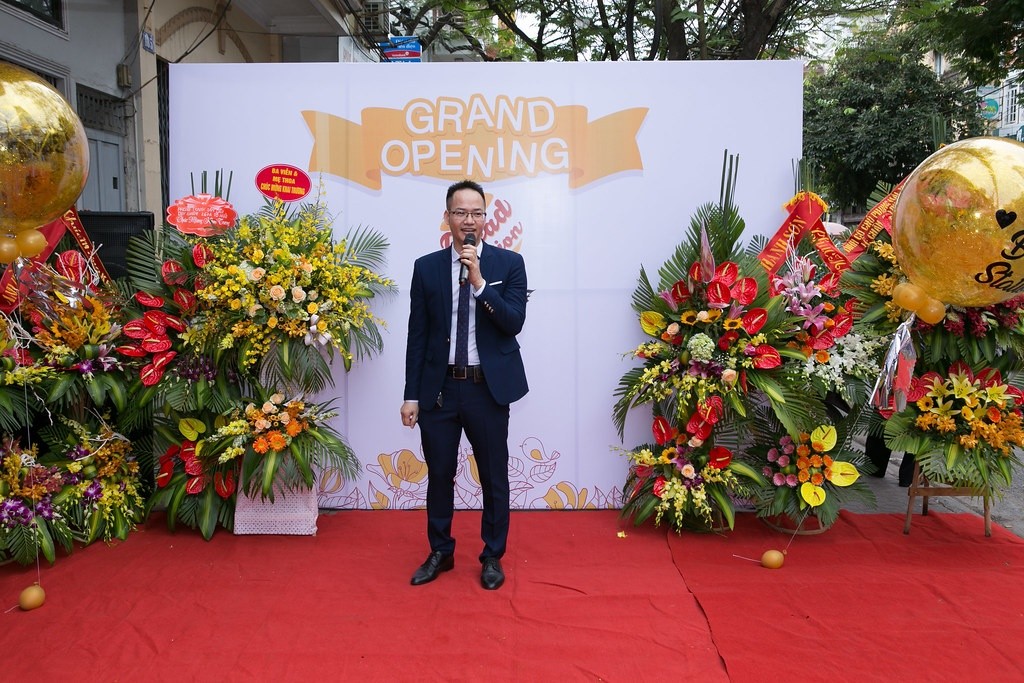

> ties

[{"left": 453, "top": 254, "right": 479, "bottom": 371}]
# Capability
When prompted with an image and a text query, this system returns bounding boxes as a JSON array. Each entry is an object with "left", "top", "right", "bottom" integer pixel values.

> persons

[
  {"left": 860, "top": 410, "right": 925, "bottom": 487},
  {"left": 401, "top": 180, "right": 529, "bottom": 590}
]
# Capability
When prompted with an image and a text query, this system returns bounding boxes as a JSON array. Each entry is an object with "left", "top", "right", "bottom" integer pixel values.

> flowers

[
  {"left": 0, "top": 168, "right": 403, "bottom": 566},
  {"left": 611, "top": 113, "right": 1023, "bottom": 538}
]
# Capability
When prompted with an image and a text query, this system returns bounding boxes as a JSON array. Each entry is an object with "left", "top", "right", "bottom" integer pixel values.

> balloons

[
  {"left": 0, "top": 62, "right": 90, "bottom": 262},
  {"left": 892, "top": 136, "right": 1024, "bottom": 323}
]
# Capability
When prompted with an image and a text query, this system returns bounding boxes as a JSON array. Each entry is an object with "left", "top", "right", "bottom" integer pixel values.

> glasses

[{"left": 448, "top": 209, "right": 487, "bottom": 220}]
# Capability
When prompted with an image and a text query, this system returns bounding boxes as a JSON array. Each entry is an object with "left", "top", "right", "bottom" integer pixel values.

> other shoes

[
  {"left": 898, "top": 479, "right": 913, "bottom": 488},
  {"left": 857, "top": 463, "right": 885, "bottom": 479}
]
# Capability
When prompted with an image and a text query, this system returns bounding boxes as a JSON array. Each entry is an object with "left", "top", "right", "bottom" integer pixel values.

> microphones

[{"left": 459, "top": 233, "right": 476, "bottom": 287}]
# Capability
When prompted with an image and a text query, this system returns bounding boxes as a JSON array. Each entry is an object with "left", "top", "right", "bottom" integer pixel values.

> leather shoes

[
  {"left": 479, "top": 558, "right": 505, "bottom": 590},
  {"left": 409, "top": 550, "right": 455, "bottom": 586}
]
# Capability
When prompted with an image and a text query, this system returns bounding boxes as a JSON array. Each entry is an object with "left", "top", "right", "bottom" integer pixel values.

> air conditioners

[{"left": 357, "top": 1, "right": 391, "bottom": 33}]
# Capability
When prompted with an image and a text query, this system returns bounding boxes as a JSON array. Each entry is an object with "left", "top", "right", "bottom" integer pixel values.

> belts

[{"left": 446, "top": 365, "right": 474, "bottom": 380}]
